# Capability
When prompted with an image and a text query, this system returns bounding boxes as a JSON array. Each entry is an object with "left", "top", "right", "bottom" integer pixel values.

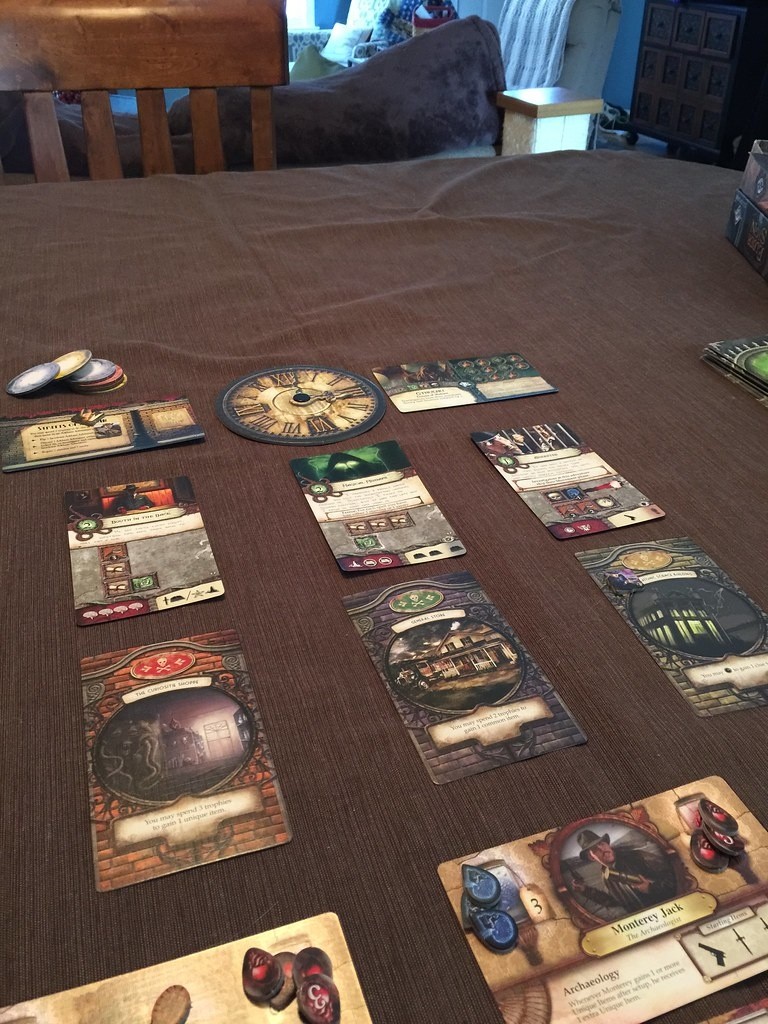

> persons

[
  {"left": 470, "top": 424, "right": 562, "bottom": 457},
  {"left": 113, "top": 485, "right": 153, "bottom": 516},
  {"left": 573, "top": 829, "right": 676, "bottom": 915}
]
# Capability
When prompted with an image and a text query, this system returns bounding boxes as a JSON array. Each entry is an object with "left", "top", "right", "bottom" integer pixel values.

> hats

[{"left": 577, "top": 830, "right": 610, "bottom": 860}]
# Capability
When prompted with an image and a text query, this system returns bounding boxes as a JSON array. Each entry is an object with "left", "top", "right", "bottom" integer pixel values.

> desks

[{"left": 0, "top": 150, "right": 768, "bottom": 1024}]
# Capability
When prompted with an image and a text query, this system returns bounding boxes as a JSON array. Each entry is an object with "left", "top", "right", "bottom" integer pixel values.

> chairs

[{"left": 0, "top": 0, "right": 290, "bottom": 184}]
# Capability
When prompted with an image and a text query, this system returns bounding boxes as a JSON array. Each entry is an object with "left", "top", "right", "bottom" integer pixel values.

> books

[{"left": 700, "top": 335, "right": 768, "bottom": 406}]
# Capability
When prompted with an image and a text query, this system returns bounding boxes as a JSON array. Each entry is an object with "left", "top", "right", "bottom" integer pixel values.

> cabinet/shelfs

[{"left": 626, "top": 0, "right": 768, "bottom": 168}]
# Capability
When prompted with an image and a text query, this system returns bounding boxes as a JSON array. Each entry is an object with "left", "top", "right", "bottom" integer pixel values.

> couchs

[
  {"left": 0, "top": 16, "right": 506, "bottom": 178},
  {"left": 287, "top": 0, "right": 622, "bottom": 150}
]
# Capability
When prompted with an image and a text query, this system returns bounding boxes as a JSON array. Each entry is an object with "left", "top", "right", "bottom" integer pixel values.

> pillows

[
  {"left": 290, "top": 44, "right": 348, "bottom": 81},
  {"left": 320, "top": 21, "right": 372, "bottom": 65}
]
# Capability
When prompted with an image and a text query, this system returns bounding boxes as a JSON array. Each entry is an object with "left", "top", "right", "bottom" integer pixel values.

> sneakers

[{"left": 599, "top": 102, "right": 630, "bottom": 135}]
